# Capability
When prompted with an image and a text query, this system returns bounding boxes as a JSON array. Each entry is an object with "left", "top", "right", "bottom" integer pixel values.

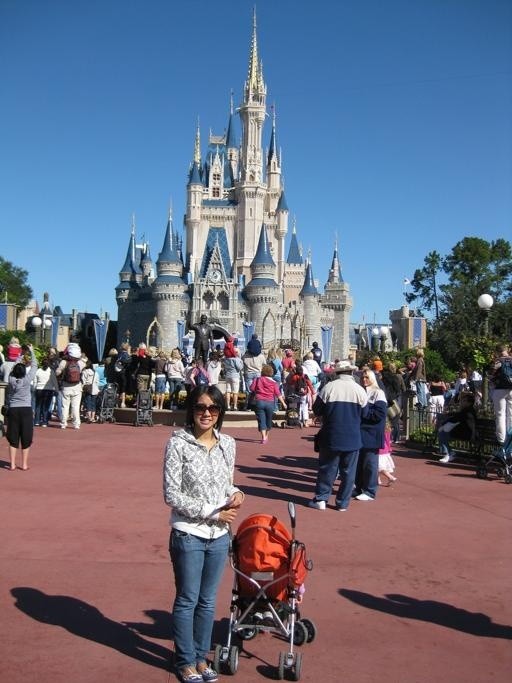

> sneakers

[
  {"left": 439, "top": 455, "right": 454, "bottom": 464},
  {"left": 308, "top": 488, "right": 375, "bottom": 511},
  {"left": 196, "top": 662, "right": 218, "bottom": 682},
  {"left": 174, "top": 664, "right": 204, "bottom": 683}
]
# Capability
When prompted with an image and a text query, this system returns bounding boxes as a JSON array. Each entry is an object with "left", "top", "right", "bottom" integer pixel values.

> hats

[{"left": 334, "top": 361, "right": 359, "bottom": 373}]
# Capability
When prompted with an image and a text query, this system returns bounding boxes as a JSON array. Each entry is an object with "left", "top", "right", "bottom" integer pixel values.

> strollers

[
  {"left": 98, "top": 382, "right": 117, "bottom": 422},
  {"left": 476, "top": 427, "right": 512, "bottom": 483},
  {"left": 211, "top": 500, "right": 317, "bottom": 680},
  {"left": 281, "top": 393, "right": 304, "bottom": 428},
  {"left": 133, "top": 387, "right": 154, "bottom": 427}
]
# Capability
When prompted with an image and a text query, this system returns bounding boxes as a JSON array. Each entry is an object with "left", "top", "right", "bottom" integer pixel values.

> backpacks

[
  {"left": 295, "top": 375, "right": 307, "bottom": 396},
  {"left": 63, "top": 360, "right": 80, "bottom": 383},
  {"left": 269, "top": 359, "right": 280, "bottom": 375},
  {"left": 496, "top": 357, "right": 512, "bottom": 389}
]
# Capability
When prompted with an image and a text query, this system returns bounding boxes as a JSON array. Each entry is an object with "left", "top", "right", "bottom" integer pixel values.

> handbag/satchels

[
  {"left": 1, "top": 406, "right": 9, "bottom": 415},
  {"left": 83, "top": 385, "right": 92, "bottom": 394},
  {"left": 248, "top": 399, "right": 257, "bottom": 409},
  {"left": 196, "top": 372, "right": 208, "bottom": 386}
]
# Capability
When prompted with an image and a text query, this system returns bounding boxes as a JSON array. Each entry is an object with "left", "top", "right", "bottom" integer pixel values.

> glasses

[{"left": 192, "top": 403, "right": 221, "bottom": 417}]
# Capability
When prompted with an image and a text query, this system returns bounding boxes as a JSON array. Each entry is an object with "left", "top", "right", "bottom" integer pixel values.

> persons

[
  {"left": 295, "top": 583, "right": 305, "bottom": 604},
  {"left": 183, "top": 309, "right": 216, "bottom": 364},
  {"left": 306, "top": 360, "right": 370, "bottom": 512},
  {"left": 4, "top": 344, "right": 40, "bottom": 472},
  {"left": 350, "top": 368, "right": 389, "bottom": 502},
  {"left": 322, "top": 343, "right": 511, "bottom": 487},
  {"left": 161, "top": 382, "right": 247, "bottom": 682},
  {"left": 0, "top": 336, "right": 321, "bottom": 445}
]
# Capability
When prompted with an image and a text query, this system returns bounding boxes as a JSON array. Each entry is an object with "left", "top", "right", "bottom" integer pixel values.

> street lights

[
  {"left": 371, "top": 327, "right": 379, "bottom": 352},
  {"left": 31, "top": 315, "right": 42, "bottom": 346},
  {"left": 381, "top": 325, "right": 389, "bottom": 350},
  {"left": 43, "top": 319, "right": 52, "bottom": 342},
  {"left": 477, "top": 294, "right": 496, "bottom": 413}
]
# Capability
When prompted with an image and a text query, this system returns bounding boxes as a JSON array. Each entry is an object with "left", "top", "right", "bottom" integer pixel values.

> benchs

[{"left": 419, "top": 412, "right": 499, "bottom": 465}]
